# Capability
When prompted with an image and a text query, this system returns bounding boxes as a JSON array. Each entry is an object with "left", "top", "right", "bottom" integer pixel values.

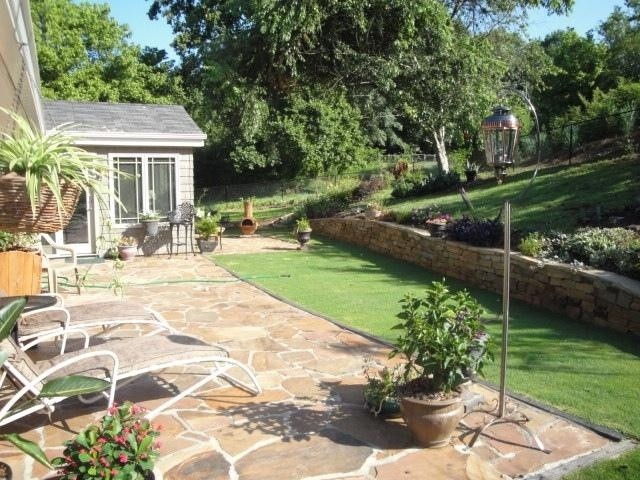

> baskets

[{"left": 0, "top": 174, "right": 82, "bottom": 234}]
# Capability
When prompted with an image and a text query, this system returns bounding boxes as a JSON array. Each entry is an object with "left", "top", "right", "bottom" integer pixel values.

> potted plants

[
  {"left": 296, "top": 216, "right": 312, "bottom": 241},
  {"left": 194, "top": 206, "right": 222, "bottom": 251},
  {"left": 361, "top": 277, "right": 493, "bottom": 450},
  {"left": 52, "top": 396, "right": 165, "bottom": 480},
  {"left": 118, "top": 208, "right": 160, "bottom": 261},
  {"left": 0, "top": 104, "right": 131, "bottom": 299}
]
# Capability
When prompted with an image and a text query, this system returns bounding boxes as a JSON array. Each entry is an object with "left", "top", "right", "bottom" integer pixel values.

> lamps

[{"left": 459, "top": 85, "right": 554, "bottom": 456}]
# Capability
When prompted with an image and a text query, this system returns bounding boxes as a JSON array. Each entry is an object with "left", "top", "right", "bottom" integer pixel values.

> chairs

[
  {"left": 38, "top": 232, "right": 86, "bottom": 297},
  {"left": 0, "top": 293, "right": 265, "bottom": 426},
  {"left": 167, "top": 201, "right": 197, "bottom": 260}
]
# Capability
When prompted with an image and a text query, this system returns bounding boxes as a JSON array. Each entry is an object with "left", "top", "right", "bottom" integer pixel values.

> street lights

[{"left": 479, "top": 86, "right": 542, "bottom": 419}]
